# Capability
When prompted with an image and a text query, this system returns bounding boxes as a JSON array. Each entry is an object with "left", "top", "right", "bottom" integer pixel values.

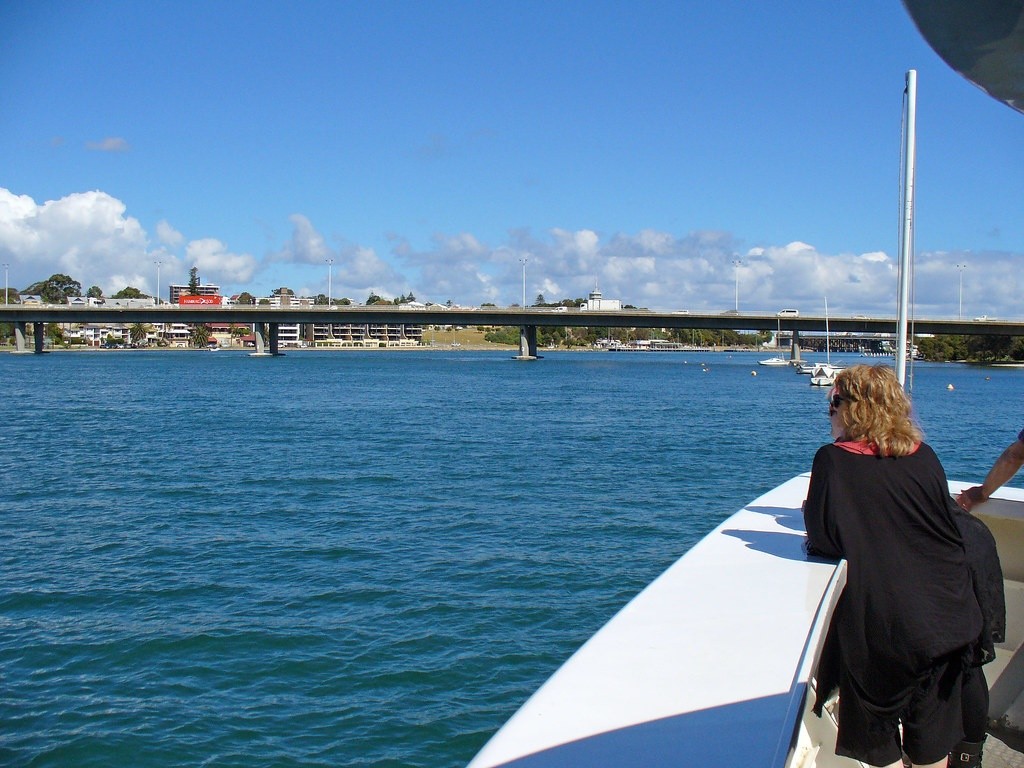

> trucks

[{"left": 580, "top": 300, "right": 621, "bottom": 311}]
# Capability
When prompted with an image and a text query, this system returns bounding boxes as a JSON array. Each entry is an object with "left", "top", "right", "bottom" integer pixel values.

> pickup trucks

[{"left": 973, "top": 315, "right": 996, "bottom": 321}]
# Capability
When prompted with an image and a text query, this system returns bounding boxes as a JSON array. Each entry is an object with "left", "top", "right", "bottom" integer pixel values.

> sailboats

[
  {"left": 757, "top": 320, "right": 790, "bottom": 366},
  {"left": 796, "top": 297, "right": 846, "bottom": 374}
]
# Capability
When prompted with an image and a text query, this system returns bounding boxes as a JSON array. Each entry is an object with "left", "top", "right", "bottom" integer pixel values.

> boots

[{"left": 951, "top": 740, "right": 985, "bottom": 768}]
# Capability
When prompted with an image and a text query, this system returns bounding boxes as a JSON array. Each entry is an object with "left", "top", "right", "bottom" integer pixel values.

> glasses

[
  {"left": 833, "top": 394, "right": 845, "bottom": 408},
  {"left": 829, "top": 407, "right": 837, "bottom": 417}
]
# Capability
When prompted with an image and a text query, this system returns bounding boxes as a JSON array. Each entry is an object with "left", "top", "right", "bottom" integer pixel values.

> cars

[
  {"left": 246, "top": 342, "right": 254, "bottom": 347},
  {"left": 776, "top": 309, "right": 798, "bottom": 317},
  {"left": 551, "top": 307, "right": 567, "bottom": 313},
  {"left": 101, "top": 344, "right": 139, "bottom": 349},
  {"left": 278, "top": 344, "right": 285, "bottom": 348},
  {"left": 207, "top": 344, "right": 215, "bottom": 347},
  {"left": 672, "top": 310, "right": 688, "bottom": 315},
  {"left": 720, "top": 310, "right": 739, "bottom": 315},
  {"left": 327, "top": 306, "right": 338, "bottom": 311},
  {"left": 851, "top": 315, "right": 870, "bottom": 320}
]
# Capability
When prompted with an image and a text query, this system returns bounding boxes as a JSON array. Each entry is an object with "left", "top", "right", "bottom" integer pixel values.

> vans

[{"left": 450, "top": 343, "right": 461, "bottom": 347}]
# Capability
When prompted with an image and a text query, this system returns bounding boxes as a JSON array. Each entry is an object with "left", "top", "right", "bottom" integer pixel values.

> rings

[{"left": 963, "top": 504, "right": 965, "bottom": 506}]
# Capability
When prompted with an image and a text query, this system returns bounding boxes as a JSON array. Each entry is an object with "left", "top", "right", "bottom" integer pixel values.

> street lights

[
  {"left": 3, "top": 264, "right": 9, "bottom": 305},
  {"left": 732, "top": 261, "right": 741, "bottom": 310},
  {"left": 519, "top": 259, "right": 527, "bottom": 310},
  {"left": 154, "top": 262, "right": 162, "bottom": 305},
  {"left": 957, "top": 265, "right": 966, "bottom": 320},
  {"left": 326, "top": 259, "right": 333, "bottom": 308}
]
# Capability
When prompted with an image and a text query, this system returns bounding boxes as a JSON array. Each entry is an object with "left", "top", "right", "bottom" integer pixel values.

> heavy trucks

[{"left": 179, "top": 295, "right": 234, "bottom": 308}]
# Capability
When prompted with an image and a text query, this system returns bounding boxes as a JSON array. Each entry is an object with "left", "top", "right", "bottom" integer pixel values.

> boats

[{"left": 810, "top": 367, "right": 837, "bottom": 386}]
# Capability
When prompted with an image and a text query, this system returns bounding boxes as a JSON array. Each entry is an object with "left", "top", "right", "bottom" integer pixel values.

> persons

[
  {"left": 804, "top": 361, "right": 1006, "bottom": 767},
  {"left": 959, "top": 430, "right": 1024, "bottom": 513}
]
